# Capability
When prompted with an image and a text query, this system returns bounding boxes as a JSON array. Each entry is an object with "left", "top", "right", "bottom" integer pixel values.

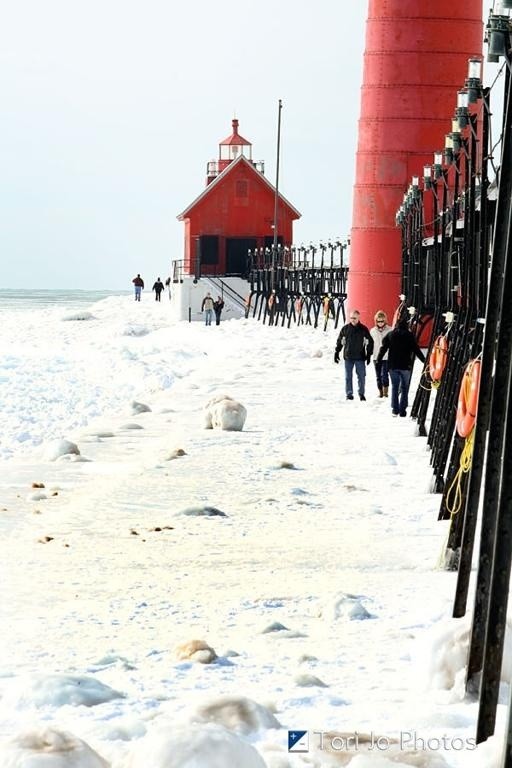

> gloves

[
  {"left": 365, "top": 355, "right": 370, "bottom": 365},
  {"left": 334, "top": 352, "right": 340, "bottom": 363}
]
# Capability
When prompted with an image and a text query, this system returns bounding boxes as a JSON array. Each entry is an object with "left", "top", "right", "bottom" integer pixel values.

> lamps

[
  {"left": 393, "top": 0, "right": 512, "bottom": 226},
  {"left": 247, "top": 235, "right": 351, "bottom": 256}
]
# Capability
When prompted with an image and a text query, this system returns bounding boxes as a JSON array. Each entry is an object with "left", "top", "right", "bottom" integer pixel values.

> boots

[{"left": 378, "top": 386, "right": 388, "bottom": 397}]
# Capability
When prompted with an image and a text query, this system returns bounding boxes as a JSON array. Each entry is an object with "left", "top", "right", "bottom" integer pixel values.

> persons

[
  {"left": 334, "top": 310, "right": 374, "bottom": 401},
  {"left": 132, "top": 274, "right": 145, "bottom": 301},
  {"left": 214, "top": 296, "right": 225, "bottom": 325},
  {"left": 152, "top": 277, "right": 165, "bottom": 302},
  {"left": 363, "top": 310, "right": 395, "bottom": 399},
  {"left": 200, "top": 292, "right": 215, "bottom": 326},
  {"left": 376, "top": 318, "right": 425, "bottom": 417}
]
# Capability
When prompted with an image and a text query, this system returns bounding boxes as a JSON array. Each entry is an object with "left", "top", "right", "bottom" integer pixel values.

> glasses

[
  {"left": 351, "top": 318, "right": 357, "bottom": 319},
  {"left": 377, "top": 320, "right": 384, "bottom": 322}
]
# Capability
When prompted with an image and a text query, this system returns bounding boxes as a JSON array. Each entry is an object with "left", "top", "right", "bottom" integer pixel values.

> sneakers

[
  {"left": 392, "top": 409, "right": 407, "bottom": 417},
  {"left": 347, "top": 395, "right": 353, "bottom": 400},
  {"left": 359, "top": 394, "right": 366, "bottom": 402}
]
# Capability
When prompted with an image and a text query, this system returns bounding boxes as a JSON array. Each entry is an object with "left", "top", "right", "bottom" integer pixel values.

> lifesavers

[
  {"left": 430, "top": 335, "right": 448, "bottom": 381},
  {"left": 456, "top": 359, "right": 480, "bottom": 437}
]
